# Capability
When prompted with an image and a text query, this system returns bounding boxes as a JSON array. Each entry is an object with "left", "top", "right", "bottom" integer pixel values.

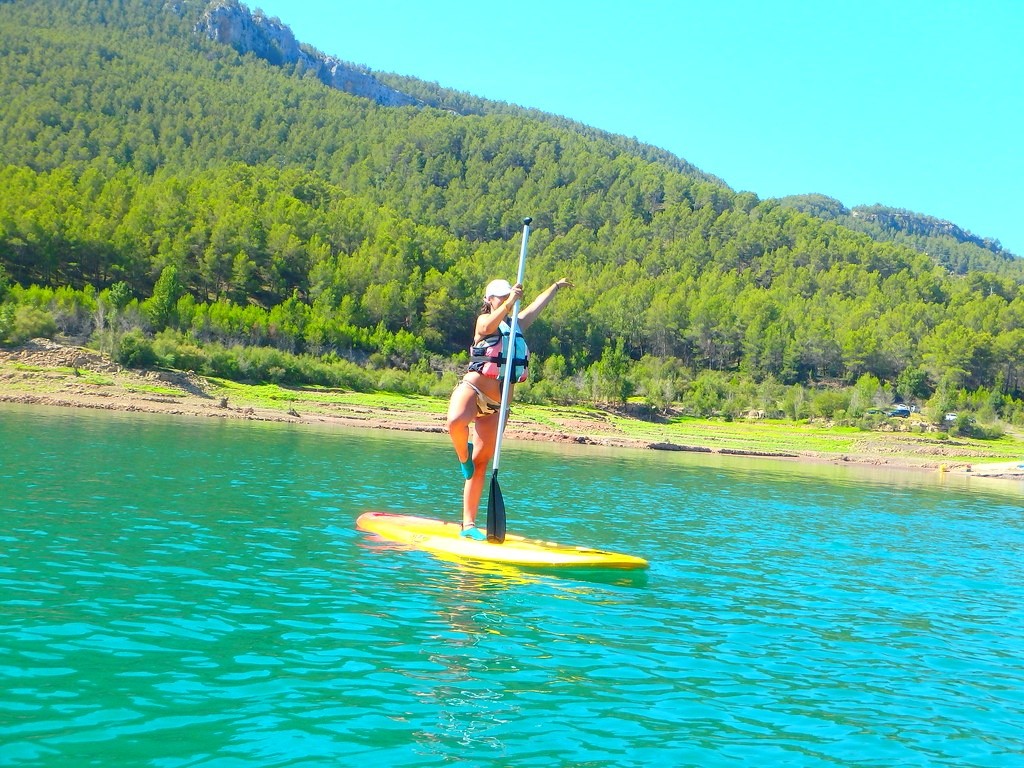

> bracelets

[
  {"left": 555, "top": 282, "right": 559, "bottom": 292},
  {"left": 504, "top": 301, "right": 510, "bottom": 313}
]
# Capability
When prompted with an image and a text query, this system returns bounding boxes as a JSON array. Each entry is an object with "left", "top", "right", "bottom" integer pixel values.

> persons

[{"left": 447, "top": 278, "right": 575, "bottom": 541}]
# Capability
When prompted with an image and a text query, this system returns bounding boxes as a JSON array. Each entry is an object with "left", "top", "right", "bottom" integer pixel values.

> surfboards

[{"left": 357, "top": 510, "right": 649, "bottom": 570}]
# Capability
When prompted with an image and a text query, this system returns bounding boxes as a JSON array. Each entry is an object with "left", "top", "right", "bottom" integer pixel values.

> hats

[{"left": 486, "top": 279, "right": 511, "bottom": 298}]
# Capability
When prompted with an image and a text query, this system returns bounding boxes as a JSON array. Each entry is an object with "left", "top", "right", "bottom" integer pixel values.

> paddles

[{"left": 487, "top": 217, "right": 535, "bottom": 543}]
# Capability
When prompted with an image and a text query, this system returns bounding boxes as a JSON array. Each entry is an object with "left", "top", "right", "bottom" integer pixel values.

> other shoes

[
  {"left": 461, "top": 442, "right": 475, "bottom": 479},
  {"left": 460, "top": 526, "right": 486, "bottom": 540}
]
